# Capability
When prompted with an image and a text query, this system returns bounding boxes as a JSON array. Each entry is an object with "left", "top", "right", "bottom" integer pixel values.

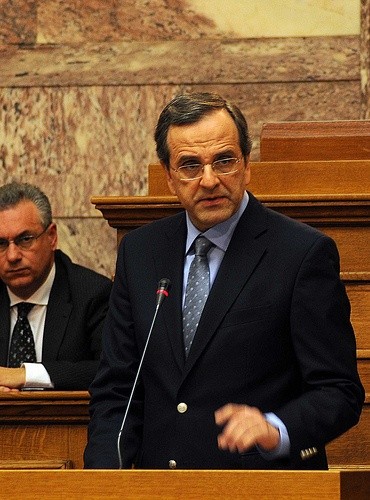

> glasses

[
  {"left": 169, "top": 154, "right": 244, "bottom": 181},
  {"left": 0, "top": 222, "right": 53, "bottom": 253}
]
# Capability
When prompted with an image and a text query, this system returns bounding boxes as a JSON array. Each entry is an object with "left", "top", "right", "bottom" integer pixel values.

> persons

[
  {"left": 84, "top": 92, "right": 365, "bottom": 471},
  {"left": 0, "top": 182, "right": 114, "bottom": 394}
]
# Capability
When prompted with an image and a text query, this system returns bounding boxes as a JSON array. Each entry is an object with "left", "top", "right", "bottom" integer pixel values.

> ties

[
  {"left": 8, "top": 303, "right": 37, "bottom": 368},
  {"left": 183, "top": 236, "right": 213, "bottom": 360}
]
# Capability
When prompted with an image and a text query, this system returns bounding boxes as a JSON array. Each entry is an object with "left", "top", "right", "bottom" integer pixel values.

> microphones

[{"left": 117, "top": 277, "right": 171, "bottom": 469}]
